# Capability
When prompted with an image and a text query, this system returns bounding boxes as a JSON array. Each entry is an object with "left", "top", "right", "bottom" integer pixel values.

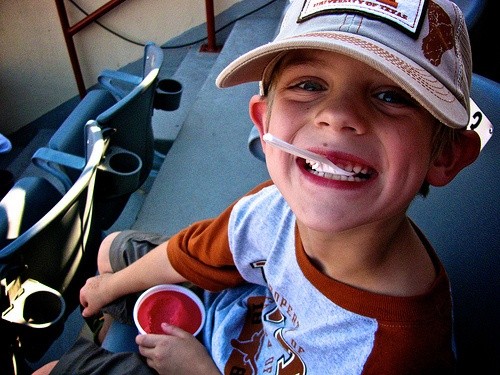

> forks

[{"left": 262, "top": 133, "right": 354, "bottom": 176}]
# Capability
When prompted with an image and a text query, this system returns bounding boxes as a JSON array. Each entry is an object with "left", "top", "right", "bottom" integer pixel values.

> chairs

[
  {"left": 248, "top": 0, "right": 500, "bottom": 375},
  {"left": 0, "top": 42, "right": 184, "bottom": 375}
]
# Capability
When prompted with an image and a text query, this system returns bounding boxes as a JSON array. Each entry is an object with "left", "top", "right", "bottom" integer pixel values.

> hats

[{"left": 215, "top": 0, "right": 473, "bottom": 128}]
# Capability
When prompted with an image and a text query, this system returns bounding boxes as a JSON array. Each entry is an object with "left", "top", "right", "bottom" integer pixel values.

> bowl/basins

[{"left": 133, "top": 284, "right": 205, "bottom": 337}]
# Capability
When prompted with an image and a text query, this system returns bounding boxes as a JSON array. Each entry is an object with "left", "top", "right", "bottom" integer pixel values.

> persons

[{"left": 31, "top": 0, "right": 482, "bottom": 375}]
